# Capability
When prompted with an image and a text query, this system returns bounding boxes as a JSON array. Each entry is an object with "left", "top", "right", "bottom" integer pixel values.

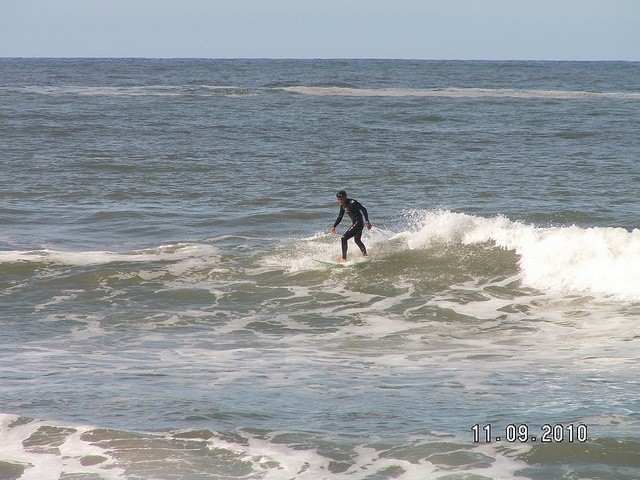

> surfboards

[{"left": 311, "top": 258, "right": 363, "bottom": 266}]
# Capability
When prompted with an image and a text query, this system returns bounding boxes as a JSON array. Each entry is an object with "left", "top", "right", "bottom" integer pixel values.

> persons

[{"left": 330, "top": 191, "right": 372, "bottom": 262}]
